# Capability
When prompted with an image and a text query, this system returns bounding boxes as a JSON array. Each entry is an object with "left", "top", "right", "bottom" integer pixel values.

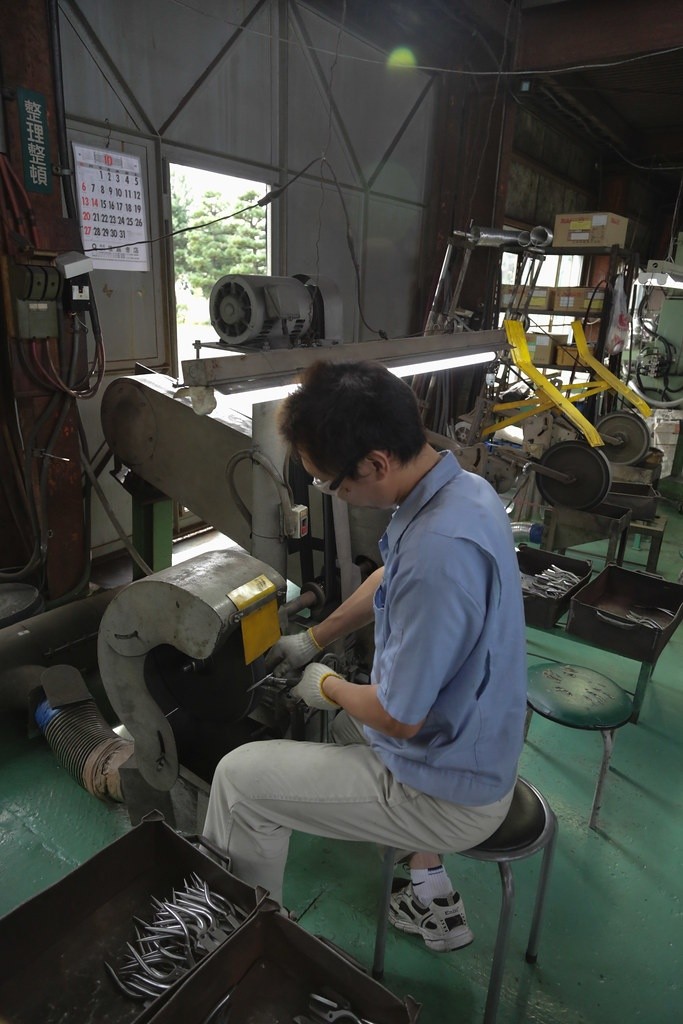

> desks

[{"left": 524, "top": 610, "right": 658, "bottom": 723}]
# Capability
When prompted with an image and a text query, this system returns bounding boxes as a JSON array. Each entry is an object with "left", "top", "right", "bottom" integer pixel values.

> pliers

[{"left": 244, "top": 667, "right": 303, "bottom": 693}]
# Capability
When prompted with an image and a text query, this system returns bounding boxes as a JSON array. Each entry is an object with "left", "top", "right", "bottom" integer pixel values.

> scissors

[
  {"left": 103, "top": 871, "right": 249, "bottom": 1003},
  {"left": 292, "top": 985, "right": 374, "bottom": 1024},
  {"left": 625, "top": 598, "right": 676, "bottom": 630},
  {"left": 528, "top": 564, "right": 582, "bottom": 599}
]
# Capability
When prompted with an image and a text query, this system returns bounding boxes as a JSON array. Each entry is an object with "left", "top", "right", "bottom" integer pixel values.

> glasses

[{"left": 312, "top": 446, "right": 385, "bottom": 496}]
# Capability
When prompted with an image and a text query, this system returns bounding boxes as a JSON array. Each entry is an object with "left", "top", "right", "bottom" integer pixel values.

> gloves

[
  {"left": 289, "top": 662, "right": 347, "bottom": 710},
  {"left": 270, "top": 627, "right": 323, "bottom": 678}
]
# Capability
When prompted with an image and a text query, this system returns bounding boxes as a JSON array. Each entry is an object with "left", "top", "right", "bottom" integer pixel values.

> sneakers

[{"left": 387, "top": 862, "right": 474, "bottom": 951}]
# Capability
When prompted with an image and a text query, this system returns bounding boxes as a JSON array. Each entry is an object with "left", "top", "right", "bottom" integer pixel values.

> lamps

[{"left": 171, "top": 328, "right": 517, "bottom": 417}]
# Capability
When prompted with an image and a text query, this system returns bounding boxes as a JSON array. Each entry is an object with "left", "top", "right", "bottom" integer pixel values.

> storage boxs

[
  {"left": 552, "top": 213, "right": 629, "bottom": 248},
  {"left": 523, "top": 333, "right": 567, "bottom": 366},
  {"left": 555, "top": 344, "right": 595, "bottom": 367},
  {"left": 498, "top": 284, "right": 554, "bottom": 310},
  {"left": 605, "top": 481, "right": 661, "bottom": 522},
  {"left": 516, "top": 542, "right": 592, "bottom": 629},
  {"left": 565, "top": 561, "right": 683, "bottom": 663},
  {"left": 0, "top": 810, "right": 269, "bottom": 1024},
  {"left": 553, "top": 286, "right": 605, "bottom": 312},
  {"left": 150, "top": 897, "right": 422, "bottom": 1024}
]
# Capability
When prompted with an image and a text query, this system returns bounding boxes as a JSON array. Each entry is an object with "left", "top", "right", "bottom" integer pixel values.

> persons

[{"left": 199, "top": 360, "right": 528, "bottom": 952}]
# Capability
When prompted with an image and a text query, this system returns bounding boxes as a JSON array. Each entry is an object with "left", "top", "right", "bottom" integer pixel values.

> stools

[
  {"left": 371, "top": 775, "right": 559, "bottom": 1024},
  {"left": 524, "top": 662, "right": 633, "bottom": 830},
  {"left": 615, "top": 514, "right": 667, "bottom": 575}
]
